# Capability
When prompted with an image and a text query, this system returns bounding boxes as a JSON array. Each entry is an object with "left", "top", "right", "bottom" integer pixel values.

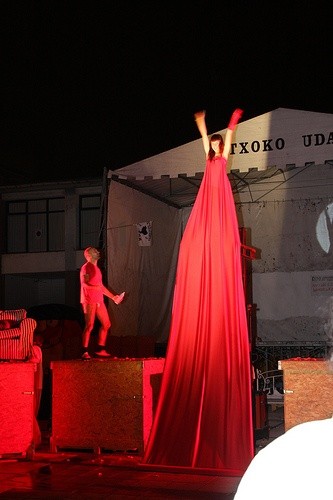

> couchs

[{"left": 0, "top": 309, "right": 38, "bottom": 362}]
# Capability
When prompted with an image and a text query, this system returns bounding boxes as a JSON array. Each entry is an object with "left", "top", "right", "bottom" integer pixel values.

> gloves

[{"left": 228, "top": 109, "right": 243, "bottom": 131}]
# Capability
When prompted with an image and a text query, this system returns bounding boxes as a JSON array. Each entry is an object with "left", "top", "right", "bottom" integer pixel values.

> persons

[
  {"left": 31, "top": 335, "right": 44, "bottom": 448},
  {"left": 38, "top": 342, "right": 52, "bottom": 431},
  {"left": 78, "top": 246, "right": 121, "bottom": 360},
  {"left": 194, "top": 107, "right": 242, "bottom": 208}
]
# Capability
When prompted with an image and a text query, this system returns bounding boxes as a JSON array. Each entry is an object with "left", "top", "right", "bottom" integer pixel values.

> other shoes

[
  {"left": 80, "top": 352, "right": 91, "bottom": 359},
  {"left": 95, "top": 350, "right": 111, "bottom": 357}
]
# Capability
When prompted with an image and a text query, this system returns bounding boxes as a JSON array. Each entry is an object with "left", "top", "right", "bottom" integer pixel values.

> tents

[{"left": 107, "top": 106, "right": 333, "bottom": 363}]
